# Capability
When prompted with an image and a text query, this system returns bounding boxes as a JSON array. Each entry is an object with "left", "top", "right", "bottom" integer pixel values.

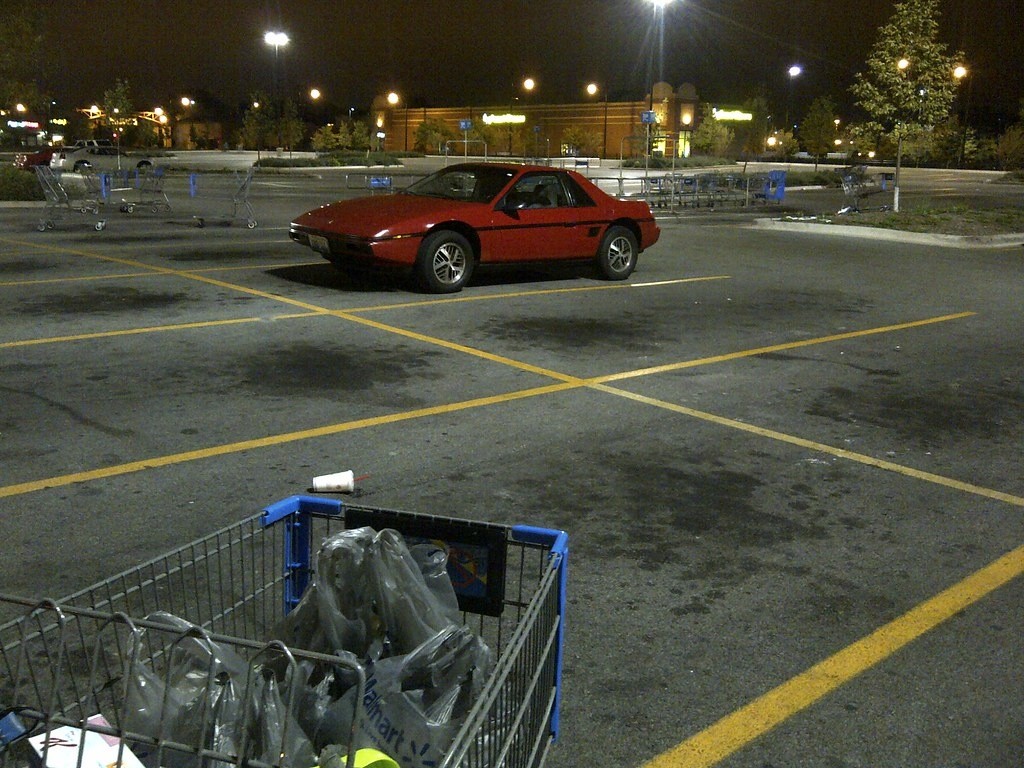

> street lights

[
  {"left": 509, "top": 79, "right": 536, "bottom": 154},
  {"left": 586, "top": 84, "right": 607, "bottom": 158},
  {"left": 387, "top": 92, "right": 409, "bottom": 152},
  {"left": 264, "top": 32, "right": 289, "bottom": 65},
  {"left": 954, "top": 67, "right": 973, "bottom": 168}
]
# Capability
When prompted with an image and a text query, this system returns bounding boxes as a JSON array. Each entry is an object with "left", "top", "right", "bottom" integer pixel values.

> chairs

[{"left": 524, "top": 184, "right": 558, "bottom": 209}]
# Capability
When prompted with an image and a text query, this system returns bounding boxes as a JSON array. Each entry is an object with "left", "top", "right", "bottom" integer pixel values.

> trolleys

[
  {"left": 650, "top": 169, "right": 787, "bottom": 208},
  {"left": 189, "top": 167, "right": 260, "bottom": 228},
  {"left": 839, "top": 173, "right": 893, "bottom": 213},
  {"left": 34, "top": 162, "right": 173, "bottom": 233},
  {"left": 0, "top": 496, "right": 568, "bottom": 767}
]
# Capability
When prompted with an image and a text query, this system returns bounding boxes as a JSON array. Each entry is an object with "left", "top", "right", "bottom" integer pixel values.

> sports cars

[{"left": 284, "top": 164, "right": 662, "bottom": 295}]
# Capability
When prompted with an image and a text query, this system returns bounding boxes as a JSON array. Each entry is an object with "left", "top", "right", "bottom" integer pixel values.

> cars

[
  {"left": 73, "top": 140, "right": 114, "bottom": 149},
  {"left": 14, "top": 146, "right": 73, "bottom": 172},
  {"left": 49, "top": 146, "right": 156, "bottom": 174}
]
